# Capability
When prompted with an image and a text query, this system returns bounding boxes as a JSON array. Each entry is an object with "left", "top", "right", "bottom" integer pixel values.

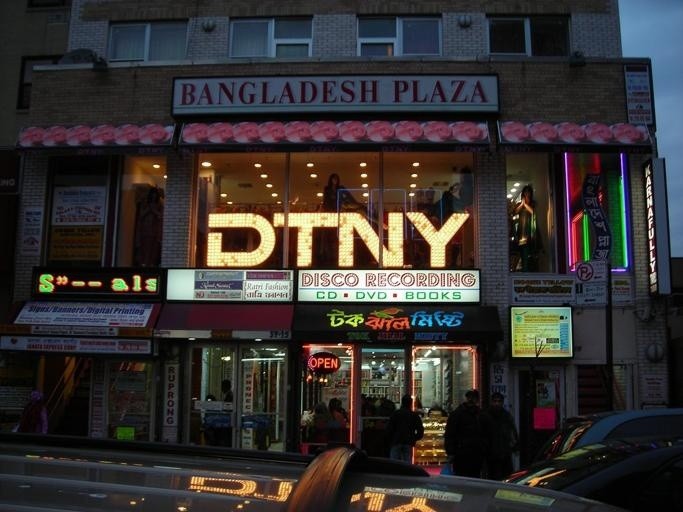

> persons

[
  {"left": 481, "top": 391, "right": 521, "bottom": 483},
  {"left": 431, "top": 182, "right": 461, "bottom": 265},
  {"left": 219, "top": 378, "right": 232, "bottom": 413},
  {"left": 510, "top": 181, "right": 536, "bottom": 272},
  {"left": 388, "top": 394, "right": 424, "bottom": 466},
  {"left": 321, "top": 170, "right": 357, "bottom": 267},
  {"left": 136, "top": 186, "right": 163, "bottom": 269},
  {"left": 11, "top": 387, "right": 49, "bottom": 437},
  {"left": 301, "top": 392, "right": 448, "bottom": 450},
  {"left": 443, "top": 388, "right": 484, "bottom": 477}
]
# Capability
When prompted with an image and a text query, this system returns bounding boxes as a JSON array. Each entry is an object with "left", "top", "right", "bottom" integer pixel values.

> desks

[{"left": 361, "top": 415, "right": 392, "bottom": 460}]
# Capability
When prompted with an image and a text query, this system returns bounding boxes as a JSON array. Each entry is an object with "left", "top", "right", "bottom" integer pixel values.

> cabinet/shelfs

[
  {"left": 321, "top": 368, "right": 424, "bottom": 410},
  {"left": 415, "top": 414, "right": 449, "bottom": 468}
]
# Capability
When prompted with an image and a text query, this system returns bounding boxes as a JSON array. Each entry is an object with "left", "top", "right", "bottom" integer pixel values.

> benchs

[{"left": 202, "top": 412, "right": 273, "bottom": 453}]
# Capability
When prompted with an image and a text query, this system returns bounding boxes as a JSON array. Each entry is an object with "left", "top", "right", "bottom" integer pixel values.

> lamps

[
  {"left": 458, "top": 13, "right": 473, "bottom": 28},
  {"left": 202, "top": 17, "right": 216, "bottom": 32},
  {"left": 645, "top": 340, "right": 666, "bottom": 363}
]
